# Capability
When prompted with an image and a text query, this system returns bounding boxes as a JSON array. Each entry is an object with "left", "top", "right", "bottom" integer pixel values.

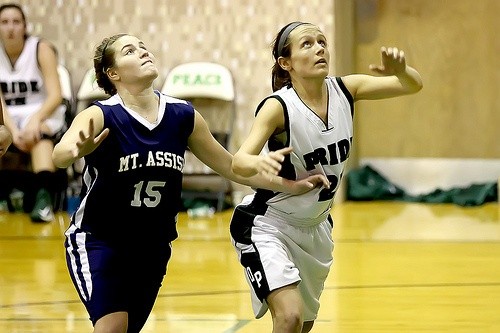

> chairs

[
  {"left": 66, "top": 68, "right": 110, "bottom": 213},
  {"left": 160, "top": 62, "right": 235, "bottom": 212}
]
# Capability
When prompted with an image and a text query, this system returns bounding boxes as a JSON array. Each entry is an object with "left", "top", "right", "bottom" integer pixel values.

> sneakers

[{"left": 31, "top": 189, "right": 54, "bottom": 222}]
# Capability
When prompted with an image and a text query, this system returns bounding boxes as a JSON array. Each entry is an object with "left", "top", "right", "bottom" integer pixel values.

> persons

[
  {"left": 51, "top": 34, "right": 330, "bottom": 333},
  {"left": 230, "top": 22, "right": 423, "bottom": 333},
  {"left": 0, "top": 5, "right": 112, "bottom": 223}
]
